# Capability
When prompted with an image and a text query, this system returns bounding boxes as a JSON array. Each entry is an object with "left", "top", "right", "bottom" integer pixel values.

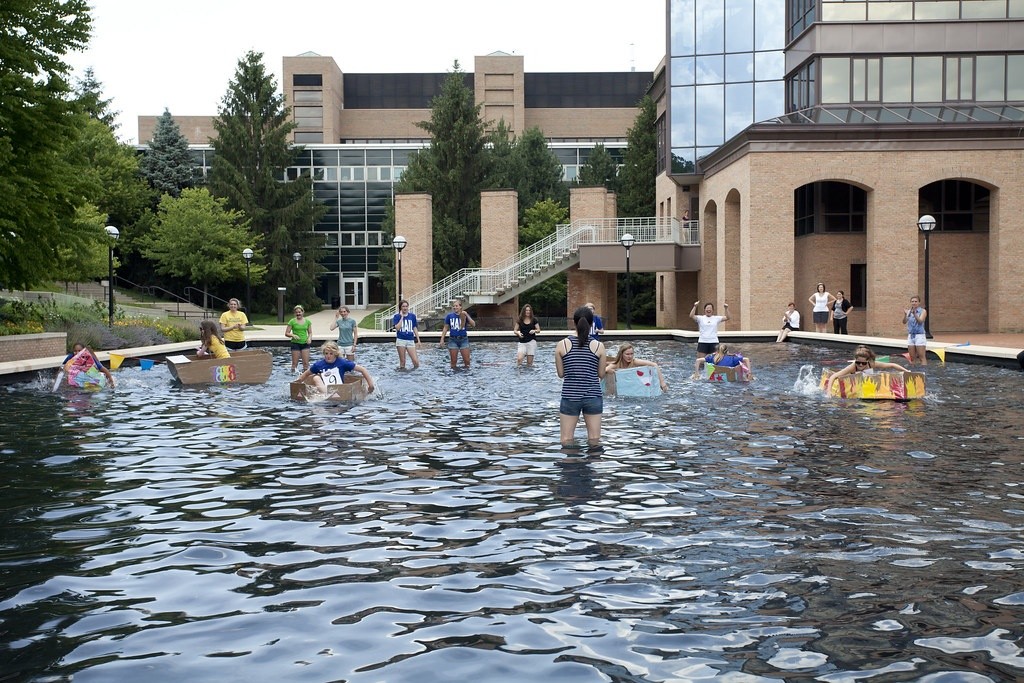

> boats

[
  {"left": 290, "top": 373, "right": 367, "bottom": 402},
  {"left": 52, "top": 345, "right": 106, "bottom": 393},
  {"left": 598, "top": 355, "right": 663, "bottom": 399},
  {"left": 818, "top": 367, "right": 927, "bottom": 400},
  {"left": 165, "top": 348, "right": 274, "bottom": 383},
  {"left": 704, "top": 361, "right": 747, "bottom": 383}
]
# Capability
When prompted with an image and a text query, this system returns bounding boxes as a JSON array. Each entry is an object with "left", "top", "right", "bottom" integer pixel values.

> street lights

[
  {"left": 392, "top": 236, "right": 407, "bottom": 314},
  {"left": 242, "top": 248, "right": 254, "bottom": 323},
  {"left": 103, "top": 225, "right": 119, "bottom": 329},
  {"left": 620, "top": 233, "right": 637, "bottom": 330},
  {"left": 916, "top": 215, "right": 936, "bottom": 340},
  {"left": 293, "top": 252, "right": 302, "bottom": 305}
]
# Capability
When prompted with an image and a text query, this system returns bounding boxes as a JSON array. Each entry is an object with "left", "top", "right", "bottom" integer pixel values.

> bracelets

[{"left": 536, "top": 330, "right": 537, "bottom": 333}]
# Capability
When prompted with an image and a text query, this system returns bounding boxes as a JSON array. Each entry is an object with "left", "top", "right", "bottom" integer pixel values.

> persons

[
  {"left": 513, "top": 304, "right": 541, "bottom": 365},
  {"left": 689, "top": 298, "right": 732, "bottom": 357},
  {"left": 828, "top": 345, "right": 911, "bottom": 390},
  {"left": 681, "top": 209, "right": 691, "bottom": 244},
  {"left": 194, "top": 320, "right": 232, "bottom": 359},
  {"left": 300, "top": 340, "right": 376, "bottom": 391},
  {"left": 830, "top": 290, "right": 854, "bottom": 334},
  {"left": 695, "top": 344, "right": 751, "bottom": 379},
  {"left": 53, "top": 342, "right": 116, "bottom": 389},
  {"left": 607, "top": 343, "right": 668, "bottom": 391},
  {"left": 285, "top": 304, "right": 313, "bottom": 372},
  {"left": 394, "top": 300, "right": 422, "bottom": 368},
  {"left": 556, "top": 302, "right": 606, "bottom": 445},
  {"left": 330, "top": 306, "right": 358, "bottom": 362},
  {"left": 440, "top": 300, "right": 475, "bottom": 369},
  {"left": 902, "top": 295, "right": 928, "bottom": 365},
  {"left": 775, "top": 303, "right": 801, "bottom": 343},
  {"left": 808, "top": 282, "right": 837, "bottom": 334},
  {"left": 219, "top": 298, "right": 248, "bottom": 351}
]
